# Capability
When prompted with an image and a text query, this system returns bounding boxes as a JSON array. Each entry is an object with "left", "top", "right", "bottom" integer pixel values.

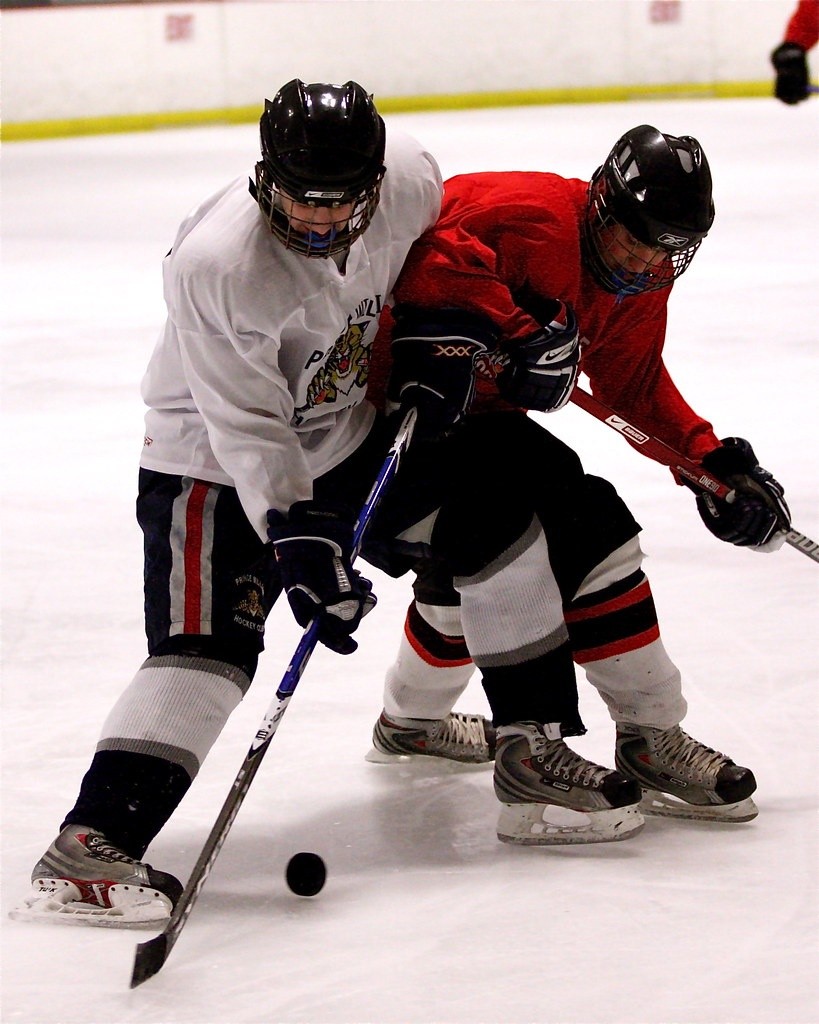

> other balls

[{"left": 285, "top": 852, "right": 327, "bottom": 898}]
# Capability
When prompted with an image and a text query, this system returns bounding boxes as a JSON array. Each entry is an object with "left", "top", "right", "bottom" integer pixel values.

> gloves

[
  {"left": 771, "top": 42, "right": 811, "bottom": 105},
  {"left": 471, "top": 296, "right": 581, "bottom": 413},
  {"left": 266, "top": 499, "right": 378, "bottom": 655},
  {"left": 680, "top": 437, "right": 792, "bottom": 553},
  {"left": 383, "top": 336, "right": 488, "bottom": 442}
]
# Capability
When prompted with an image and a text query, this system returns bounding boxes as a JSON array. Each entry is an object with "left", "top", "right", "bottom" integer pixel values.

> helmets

[
  {"left": 582, "top": 125, "right": 716, "bottom": 305},
  {"left": 248, "top": 79, "right": 386, "bottom": 259}
]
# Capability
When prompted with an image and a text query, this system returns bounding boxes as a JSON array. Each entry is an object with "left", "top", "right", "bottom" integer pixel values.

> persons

[
  {"left": 372, "top": 124, "right": 791, "bottom": 806},
  {"left": 29, "top": 78, "right": 648, "bottom": 915},
  {"left": 771, "top": 0, "right": 819, "bottom": 104}
]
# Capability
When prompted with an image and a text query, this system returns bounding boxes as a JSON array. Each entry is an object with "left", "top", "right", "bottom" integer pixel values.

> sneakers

[
  {"left": 614, "top": 720, "right": 759, "bottom": 823},
  {"left": 9, "top": 824, "right": 184, "bottom": 930},
  {"left": 364, "top": 709, "right": 496, "bottom": 772},
  {"left": 493, "top": 720, "right": 645, "bottom": 846}
]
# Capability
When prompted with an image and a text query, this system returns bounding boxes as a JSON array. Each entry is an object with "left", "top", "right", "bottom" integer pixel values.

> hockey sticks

[
  {"left": 569, "top": 383, "right": 818, "bottom": 567},
  {"left": 125, "top": 405, "right": 419, "bottom": 987}
]
